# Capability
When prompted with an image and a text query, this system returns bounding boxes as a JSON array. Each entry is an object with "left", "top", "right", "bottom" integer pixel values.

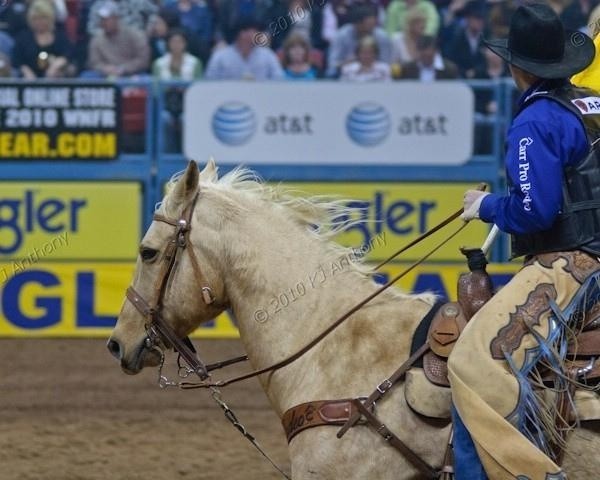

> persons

[
  {"left": 0, "top": 0, "right": 599, "bottom": 118},
  {"left": 445, "top": 0, "right": 600, "bottom": 480}
]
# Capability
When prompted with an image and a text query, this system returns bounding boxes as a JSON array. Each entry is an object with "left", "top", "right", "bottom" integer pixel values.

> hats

[{"left": 482, "top": 3, "right": 598, "bottom": 85}]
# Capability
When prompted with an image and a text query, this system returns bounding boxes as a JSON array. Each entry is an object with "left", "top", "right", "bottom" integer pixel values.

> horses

[{"left": 105, "top": 156, "right": 600, "bottom": 480}]
